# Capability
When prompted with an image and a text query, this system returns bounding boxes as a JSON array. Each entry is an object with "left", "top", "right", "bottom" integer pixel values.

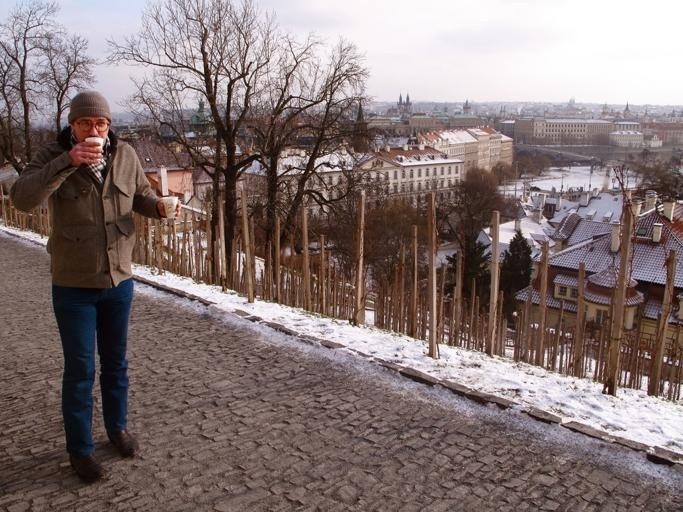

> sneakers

[
  {"left": 107, "top": 428, "right": 139, "bottom": 456},
  {"left": 68, "top": 454, "right": 106, "bottom": 484}
]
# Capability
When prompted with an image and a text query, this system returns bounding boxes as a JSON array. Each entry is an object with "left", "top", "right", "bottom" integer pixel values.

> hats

[{"left": 68, "top": 90, "right": 112, "bottom": 124}]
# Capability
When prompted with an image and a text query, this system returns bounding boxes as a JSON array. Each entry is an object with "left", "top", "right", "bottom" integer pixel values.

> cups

[
  {"left": 161, "top": 197, "right": 180, "bottom": 220},
  {"left": 84, "top": 137, "right": 103, "bottom": 156}
]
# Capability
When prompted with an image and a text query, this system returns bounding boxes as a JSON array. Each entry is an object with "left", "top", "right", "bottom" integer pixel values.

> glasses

[{"left": 74, "top": 118, "right": 110, "bottom": 132}]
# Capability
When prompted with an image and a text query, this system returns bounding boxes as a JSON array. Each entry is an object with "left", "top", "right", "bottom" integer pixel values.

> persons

[{"left": 9, "top": 91, "right": 179, "bottom": 484}]
